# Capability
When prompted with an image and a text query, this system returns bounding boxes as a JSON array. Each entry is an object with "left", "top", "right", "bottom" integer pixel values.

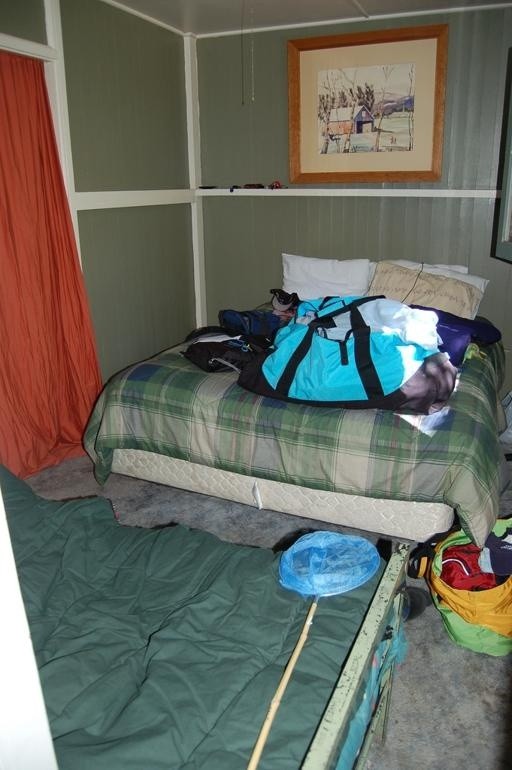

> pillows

[{"left": 281, "top": 251, "right": 489, "bottom": 319}]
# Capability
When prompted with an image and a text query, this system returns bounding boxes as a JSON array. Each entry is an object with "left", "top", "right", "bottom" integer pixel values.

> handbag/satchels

[
  {"left": 218, "top": 308, "right": 282, "bottom": 345},
  {"left": 407, "top": 517, "right": 511, "bottom": 658},
  {"left": 409, "top": 303, "right": 502, "bottom": 366},
  {"left": 236, "top": 293, "right": 458, "bottom": 415},
  {"left": 183, "top": 340, "right": 259, "bottom": 374}
]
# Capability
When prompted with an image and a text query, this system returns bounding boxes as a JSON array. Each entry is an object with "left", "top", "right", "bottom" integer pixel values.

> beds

[
  {"left": 2, "top": 463, "right": 411, "bottom": 770},
  {"left": 99, "top": 299, "right": 497, "bottom": 539}
]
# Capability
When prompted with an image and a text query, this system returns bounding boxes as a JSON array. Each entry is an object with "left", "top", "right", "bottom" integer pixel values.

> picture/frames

[{"left": 288, "top": 23, "right": 448, "bottom": 185}]
